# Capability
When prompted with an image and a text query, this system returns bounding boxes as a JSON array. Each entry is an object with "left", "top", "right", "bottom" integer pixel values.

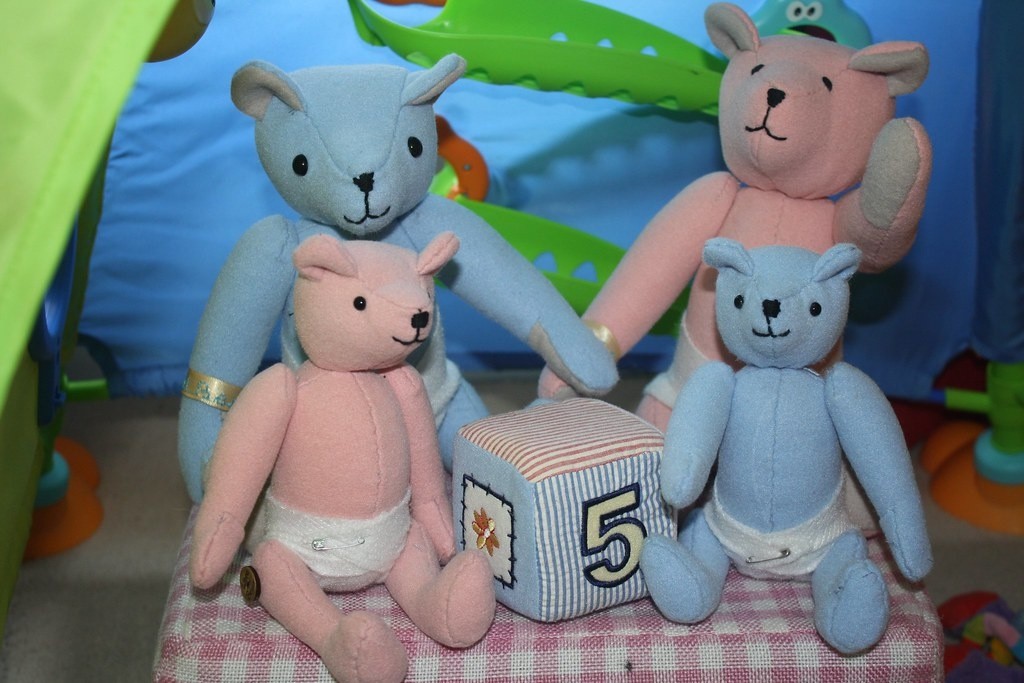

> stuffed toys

[
  {"left": 537, "top": 0, "right": 933, "bottom": 652},
  {"left": 177, "top": 55, "right": 621, "bottom": 683}
]
